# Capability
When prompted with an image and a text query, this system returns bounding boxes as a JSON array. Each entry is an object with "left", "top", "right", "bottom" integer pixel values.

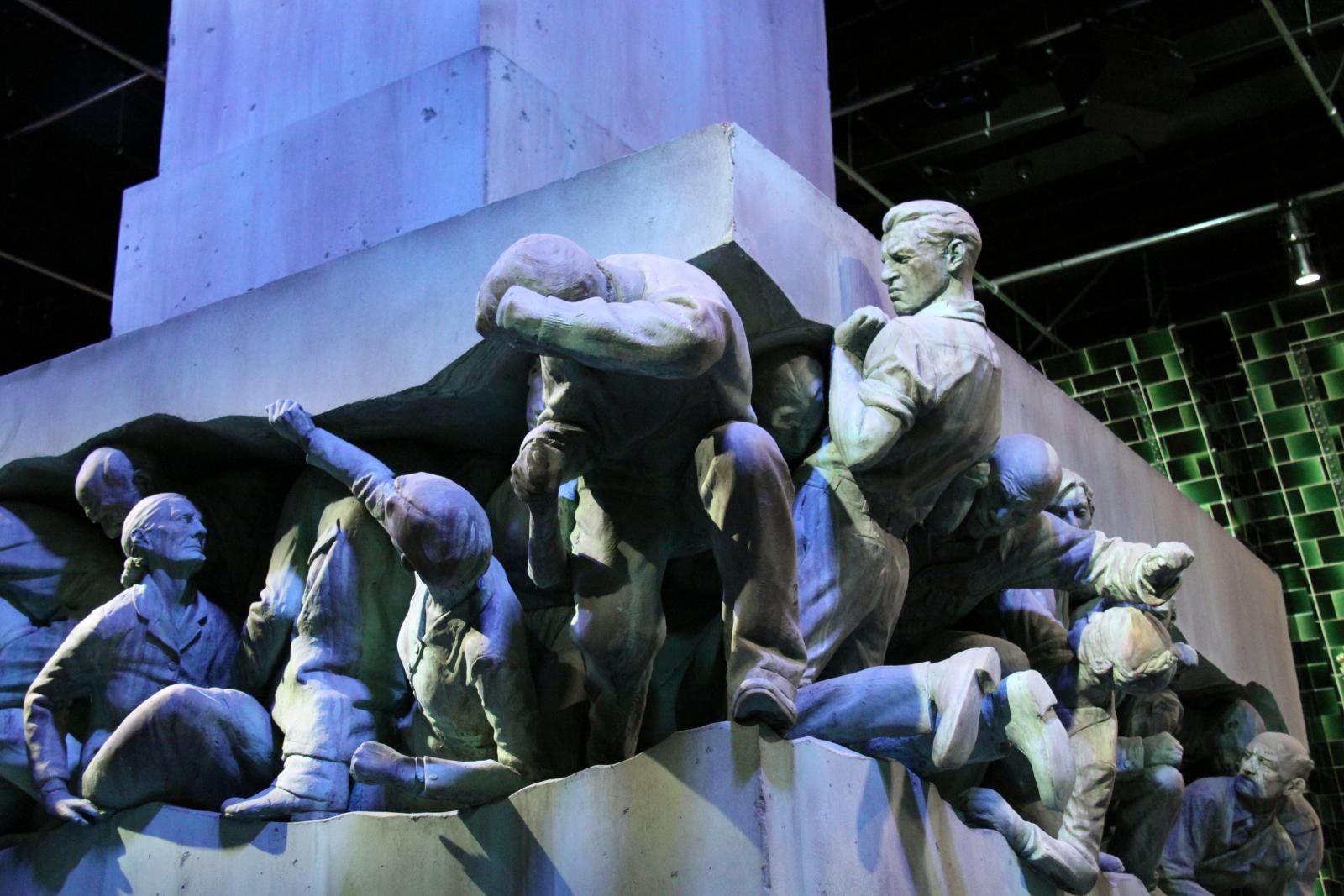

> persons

[
  {"left": 0, "top": 357, "right": 578, "bottom": 845},
  {"left": 478, "top": 234, "right": 808, "bottom": 768},
  {"left": 783, "top": 198, "right": 1076, "bottom": 808},
  {"left": 886, "top": 432, "right": 1325, "bottom": 896}
]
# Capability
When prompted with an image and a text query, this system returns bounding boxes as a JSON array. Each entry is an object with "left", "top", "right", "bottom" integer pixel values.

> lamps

[{"left": 1272, "top": 205, "right": 1325, "bottom": 289}]
